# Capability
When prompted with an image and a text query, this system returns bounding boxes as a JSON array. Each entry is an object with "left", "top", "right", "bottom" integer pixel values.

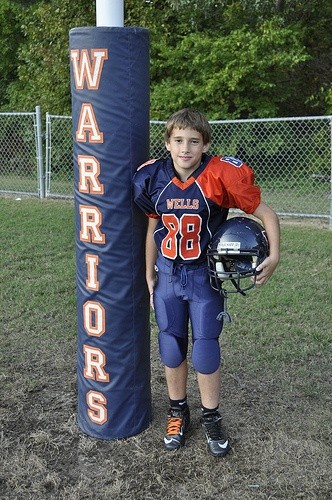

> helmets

[{"left": 206, "top": 217, "right": 270, "bottom": 279}]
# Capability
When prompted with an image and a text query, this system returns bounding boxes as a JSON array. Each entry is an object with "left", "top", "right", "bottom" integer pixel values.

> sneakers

[
  {"left": 201, "top": 411, "right": 230, "bottom": 456},
  {"left": 163, "top": 406, "right": 190, "bottom": 449}
]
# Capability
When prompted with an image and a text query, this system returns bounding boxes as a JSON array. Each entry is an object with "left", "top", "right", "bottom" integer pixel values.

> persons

[{"left": 129, "top": 108, "right": 280, "bottom": 458}]
